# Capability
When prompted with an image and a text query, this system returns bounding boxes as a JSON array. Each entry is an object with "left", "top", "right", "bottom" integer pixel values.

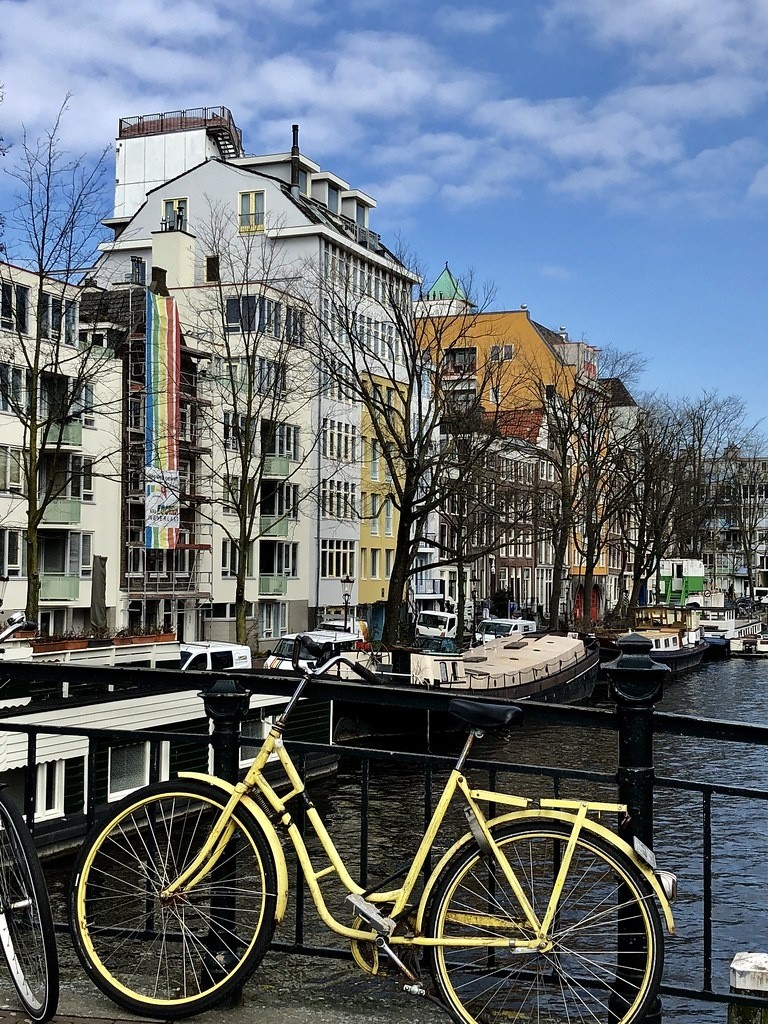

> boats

[
  {"left": 692, "top": 604, "right": 768, "bottom": 660},
  {"left": 593, "top": 604, "right": 710, "bottom": 678},
  {"left": 293, "top": 627, "right": 603, "bottom": 759}
]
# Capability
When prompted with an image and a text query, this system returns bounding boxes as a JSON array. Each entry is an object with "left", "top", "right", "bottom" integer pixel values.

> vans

[
  {"left": 264, "top": 625, "right": 365, "bottom": 676},
  {"left": 416, "top": 609, "right": 459, "bottom": 640},
  {"left": 474, "top": 616, "right": 537, "bottom": 646},
  {"left": 154, "top": 639, "right": 253, "bottom": 673}
]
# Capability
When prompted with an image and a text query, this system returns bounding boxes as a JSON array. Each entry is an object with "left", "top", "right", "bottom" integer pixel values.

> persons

[{"left": 727, "top": 583, "right": 735, "bottom": 603}]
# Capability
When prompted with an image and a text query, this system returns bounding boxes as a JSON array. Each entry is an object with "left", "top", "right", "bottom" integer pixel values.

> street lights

[
  {"left": 560, "top": 576, "right": 571, "bottom": 631},
  {"left": 468, "top": 574, "right": 481, "bottom": 649},
  {"left": 340, "top": 574, "right": 354, "bottom": 631}
]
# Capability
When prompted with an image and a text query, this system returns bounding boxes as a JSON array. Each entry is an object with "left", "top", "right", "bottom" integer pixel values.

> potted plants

[
  {"left": 62, "top": 625, "right": 89, "bottom": 650},
  {"left": 7, "top": 618, "right": 39, "bottom": 639},
  {"left": 154, "top": 621, "right": 179, "bottom": 642},
  {"left": 85, "top": 625, "right": 113, "bottom": 648},
  {"left": 131, "top": 618, "right": 157, "bottom": 644},
  {"left": 27, "top": 626, "right": 68, "bottom": 653},
  {"left": 109, "top": 625, "right": 132, "bottom": 646}
]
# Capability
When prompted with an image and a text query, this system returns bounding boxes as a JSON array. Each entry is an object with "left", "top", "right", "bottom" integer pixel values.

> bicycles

[
  {"left": 70, "top": 632, "right": 677, "bottom": 1023},
  {"left": 0, "top": 783, "right": 60, "bottom": 1024}
]
